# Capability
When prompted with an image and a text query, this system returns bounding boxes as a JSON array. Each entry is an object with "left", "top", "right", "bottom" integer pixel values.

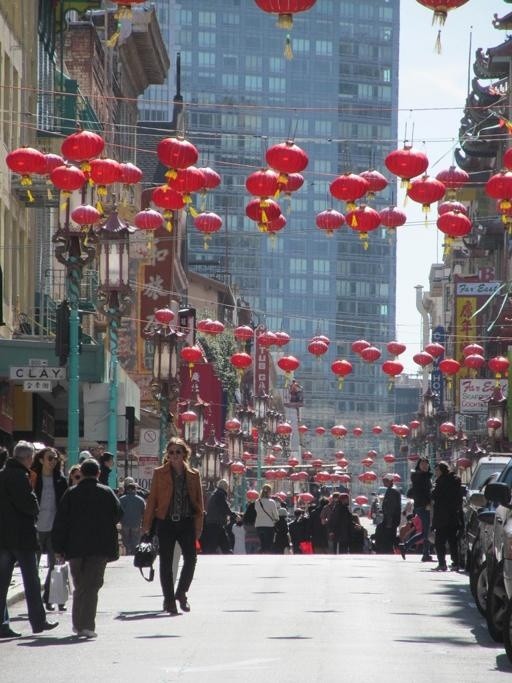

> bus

[{"left": 239, "top": 463, "right": 352, "bottom": 503}]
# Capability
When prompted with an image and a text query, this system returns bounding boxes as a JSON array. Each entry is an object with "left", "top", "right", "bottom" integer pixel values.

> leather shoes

[
  {"left": 176, "top": 591, "right": 190, "bottom": 612},
  {"left": 398, "top": 542, "right": 407, "bottom": 560},
  {"left": 422, "top": 553, "right": 466, "bottom": 571},
  {"left": 164, "top": 592, "right": 178, "bottom": 616},
  {"left": 1, "top": 592, "right": 97, "bottom": 639}
]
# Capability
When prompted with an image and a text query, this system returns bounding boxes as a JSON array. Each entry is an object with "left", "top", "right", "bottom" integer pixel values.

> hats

[{"left": 79, "top": 451, "right": 92, "bottom": 460}]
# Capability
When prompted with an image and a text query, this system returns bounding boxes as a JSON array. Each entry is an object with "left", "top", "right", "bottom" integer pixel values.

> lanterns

[
  {"left": 316, "top": 170, "right": 406, "bottom": 249},
  {"left": 387, "top": 151, "right": 473, "bottom": 252},
  {"left": 246, "top": 138, "right": 309, "bottom": 239},
  {"left": 134, "top": 138, "right": 223, "bottom": 249},
  {"left": 246, "top": 490, "right": 369, "bottom": 503},
  {"left": 486, "top": 147, "right": 512, "bottom": 232},
  {"left": 183, "top": 411, "right": 502, "bottom": 504},
  {"left": 416, "top": 0, "right": 470, "bottom": 56},
  {"left": 101, "top": 0, "right": 147, "bottom": 49},
  {"left": 5, "top": 129, "right": 143, "bottom": 244},
  {"left": 254, "top": 0, "right": 317, "bottom": 60},
  {"left": 152, "top": 308, "right": 511, "bottom": 392}
]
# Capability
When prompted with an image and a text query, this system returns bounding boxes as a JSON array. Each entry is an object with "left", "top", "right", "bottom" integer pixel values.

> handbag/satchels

[
  {"left": 134, "top": 535, "right": 159, "bottom": 566},
  {"left": 48, "top": 563, "right": 72, "bottom": 604},
  {"left": 274, "top": 518, "right": 288, "bottom": 534}
]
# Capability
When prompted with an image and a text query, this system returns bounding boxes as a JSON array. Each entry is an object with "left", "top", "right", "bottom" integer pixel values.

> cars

[
  {"left": 370, "top": 449, "right": 512, "bottom": 664},
  {"left": 350, "top": 498, "right": 372, "bottom": 517}
]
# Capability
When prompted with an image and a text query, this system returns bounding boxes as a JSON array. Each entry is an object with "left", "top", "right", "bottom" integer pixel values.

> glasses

[
  {"left": 47, "top": 455, "right": 82, "bottom": 481},
  {"left": 166, "top": 449, "right": 186, "bottom": 456}
]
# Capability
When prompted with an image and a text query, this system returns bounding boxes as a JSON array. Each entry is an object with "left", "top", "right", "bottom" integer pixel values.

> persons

[
  {"left": 49, "top": 458, "right": 124, "bottom": 637},
  {"left": 143, "top": 437, "right": 203, "bottom": 615},
  {"left": 31, "top": 448, "right": 82, "bottom": 611},
  {"left": 0, "top": 441, "right": 59, "bottom": 639},
  {"left": 0, "top": 436, "right": 466, "bottom": 571}
]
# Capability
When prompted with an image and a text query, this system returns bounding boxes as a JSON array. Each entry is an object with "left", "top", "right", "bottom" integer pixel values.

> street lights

[
  {"left": 480, "top": 379, "right": 509, "bottom": 452},
  {"left": 51, "top": 129, "right": 103, "bottom": 487},
  {"left": 410, "top": 421, "right": 420, "bottom": 465},
  {"left": 237, "top": 380, "right": 284, "bottom": 492},
  {"left": 421, "top": 379, "right": 439, "bottom": 470},
  {"left": 178, "top": 380, "right": 247, "bottom": 512},
  {"left": 91, "top": 191, "right": 143, "bottom": 489},
  {"left": 448, "top": 420, "right": 473, "bottom": 477},
  {"left": 142, "top": 304, "right": 190, "bottom": 465}
]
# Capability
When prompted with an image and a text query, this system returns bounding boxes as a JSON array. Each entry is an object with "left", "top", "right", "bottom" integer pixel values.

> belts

[{"left": 164, "top": 515, "right": 188, "bottom": 521}]
